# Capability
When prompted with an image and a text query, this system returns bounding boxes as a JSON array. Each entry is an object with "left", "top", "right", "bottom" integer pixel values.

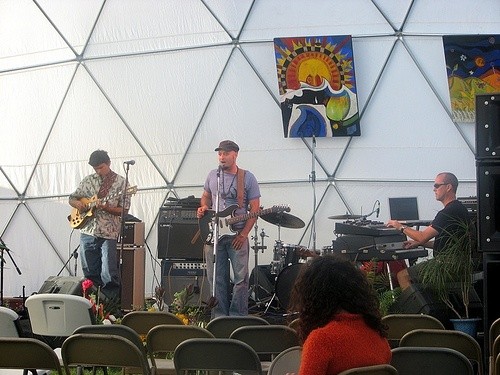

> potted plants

[{"left": 410, "top": 209, "right": 482, "bottom": 335}]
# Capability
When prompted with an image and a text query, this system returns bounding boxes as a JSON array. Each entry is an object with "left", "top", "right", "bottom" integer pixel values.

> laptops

[{"left": 389, "top": 198, "right": 434, "bottom": 223}]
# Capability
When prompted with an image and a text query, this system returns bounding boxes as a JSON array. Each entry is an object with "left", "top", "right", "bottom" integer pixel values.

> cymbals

[
  {"left": 328, "top": 215, "right": 363, "bottom": 219},
  {"left": 260, "top": 212, "right": 305, "bottom": 229}
]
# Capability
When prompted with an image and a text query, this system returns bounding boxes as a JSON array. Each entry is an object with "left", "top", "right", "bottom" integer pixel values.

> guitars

[
  {"left": 71, "top": 185, "right": 138, "bottom": 229},
  {"left": 198, "top": 203, "right": 290, "bottom": 246}
]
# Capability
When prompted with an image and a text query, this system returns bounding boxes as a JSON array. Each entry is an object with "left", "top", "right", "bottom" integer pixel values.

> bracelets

[{"left": 240, "top": 233, "right": 249, "bottom": 238}]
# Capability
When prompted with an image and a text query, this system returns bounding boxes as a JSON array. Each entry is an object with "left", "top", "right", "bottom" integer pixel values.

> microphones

[
  {"left": 124, "top": 160, "right": 135, "bottom": 165},
  {"left": 377, "top": 202, "right": 380, "bottom": 218},
  {"left": 219, "top": 165, "right": 225, "bottom": 171},
  {"left": 194, "top": 272, "right": 200, "bottom": 294}
]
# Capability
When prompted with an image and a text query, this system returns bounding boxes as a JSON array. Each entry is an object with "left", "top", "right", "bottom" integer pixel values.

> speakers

[
  {"left": 158, "top": 206, "right": 205, "bottom": 260},
  {"left": 37, "top": 275, "right": 109, "bottom": 306},
  {"left": 115, "top": 246, "right": 146, "bottom": 311},
  {"left": 161, "top": 260, "right": 211, "bottom": 306},
  {"left": 388, "top": 281, "right": 482, "bottom": 335},
  {"left": 475, "top": 93, "right": 500, "bottom": 375}
]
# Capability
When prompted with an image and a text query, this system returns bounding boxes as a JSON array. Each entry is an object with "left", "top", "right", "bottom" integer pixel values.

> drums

[
  {"left": 275, "top": 263, "right": 305, "bottom": 311},
  {"left": 296, "top": 247, "right": 320, "bottom": 263},
  {"left": 323, "top": 246, "right": 334, "bottom": 255},
  {"left": 271, "top": 244, "right": 300, "bottom": 274}
]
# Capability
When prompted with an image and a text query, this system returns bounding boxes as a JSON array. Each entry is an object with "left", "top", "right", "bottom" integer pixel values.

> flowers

[{"left": 82, "top": 279, "right": 217, "bottom": 341}]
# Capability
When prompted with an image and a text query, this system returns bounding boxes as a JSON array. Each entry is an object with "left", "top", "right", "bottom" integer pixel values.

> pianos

[{"left": 332, "top": 219, "right": 429, "bottom": 302}]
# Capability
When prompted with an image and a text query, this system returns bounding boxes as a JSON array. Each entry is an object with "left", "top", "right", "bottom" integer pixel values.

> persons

[
  {"left": 385, "top": 172, "right": 471, "bottom": 297},
  {"left": 196, "top": 141, "right": 260, "bottom": 320},
  {"left": 70, "top": 150, "right": 132, "bottom": 307},
  {"left": 285, "top": 253, "right": 392, "bottom": 375}
]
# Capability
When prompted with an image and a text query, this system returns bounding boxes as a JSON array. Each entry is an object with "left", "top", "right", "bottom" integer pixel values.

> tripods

[{"left": 247, "top": 218, "right": 278, "bottom": 311}]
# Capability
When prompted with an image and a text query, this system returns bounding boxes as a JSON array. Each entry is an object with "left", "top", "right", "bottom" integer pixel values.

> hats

[{"left": 215, "top": 140, "right": 239, "bottom": 152}]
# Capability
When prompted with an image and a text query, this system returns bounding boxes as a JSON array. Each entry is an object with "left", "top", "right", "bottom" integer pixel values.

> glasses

[{"left": 434, "top": 183, "right": 453, "bottom": 189}]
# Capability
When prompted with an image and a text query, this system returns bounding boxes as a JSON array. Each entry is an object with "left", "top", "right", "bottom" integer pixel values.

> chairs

[{"left": 0, "top": 293, "right": 500, "bottom": 375}]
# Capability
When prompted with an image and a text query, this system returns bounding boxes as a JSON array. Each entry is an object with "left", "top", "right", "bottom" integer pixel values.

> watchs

[{"left": 400, "top": 225, "right": 408, "bottom": 232}]
